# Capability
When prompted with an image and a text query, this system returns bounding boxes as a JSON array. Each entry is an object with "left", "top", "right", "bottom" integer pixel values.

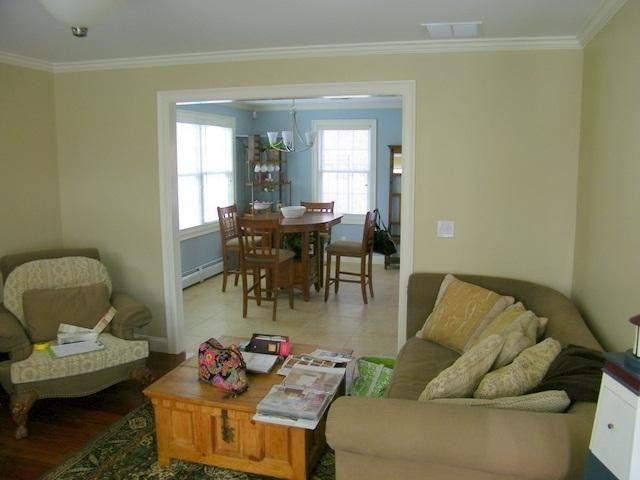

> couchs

[{"left": 325, "top": 273, "right": 606, "bottom": 480}]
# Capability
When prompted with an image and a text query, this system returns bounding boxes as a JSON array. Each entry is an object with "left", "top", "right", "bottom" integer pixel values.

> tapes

[{"left": 32, "top": 342, "right": 49, "bottom": 352}]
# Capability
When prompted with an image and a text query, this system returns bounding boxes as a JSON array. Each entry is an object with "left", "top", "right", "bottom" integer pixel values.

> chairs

[
  {"left": 0, "top": 248, "right": 162, "bottom": 441},
  {"left": 217, "top": 200, "right": 378, "bottom": 321}
]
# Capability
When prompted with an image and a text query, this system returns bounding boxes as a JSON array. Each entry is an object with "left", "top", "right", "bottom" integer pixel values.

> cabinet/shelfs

[
  {"left": 581, "top": 314, "right": 640, "bottom": 480},
  {"left": 385, "top": 145, "right": 402, "bottom": 270},
  {"left": 246, "top": 160, "right": 291, "bottom": 213}
]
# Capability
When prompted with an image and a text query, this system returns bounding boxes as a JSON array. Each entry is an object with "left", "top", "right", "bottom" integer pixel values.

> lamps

[
  {"left": 267, "top": 99, "right": 317, "bottom": 152},
  {"left": 37, "top": 0, "right": 124, "bottom": 37}
]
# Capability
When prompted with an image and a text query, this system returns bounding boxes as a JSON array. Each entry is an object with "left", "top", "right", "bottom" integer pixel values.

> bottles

[{"left": 263, "top": 173, "right": 274, "bottom": 192}]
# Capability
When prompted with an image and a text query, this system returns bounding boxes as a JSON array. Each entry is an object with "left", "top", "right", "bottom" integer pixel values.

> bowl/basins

[
  {"left": 249, "top": 200, "right": 274, "bottom": 210},
  {"left": 279, "top": 204, "right": 306, "bottom": 219}
]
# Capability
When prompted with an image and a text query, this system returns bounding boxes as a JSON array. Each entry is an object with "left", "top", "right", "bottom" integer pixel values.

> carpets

[{"left": 39, "top": 399, "right": 335, "bottom": 480}]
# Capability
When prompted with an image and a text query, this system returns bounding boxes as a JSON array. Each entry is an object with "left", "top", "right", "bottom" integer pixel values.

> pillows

[
  {"left": 23, "top": 283, "right": 112, "bottom": 344},
  {"left": 415, "top": 273, "right": 571, "bottom": 413}
]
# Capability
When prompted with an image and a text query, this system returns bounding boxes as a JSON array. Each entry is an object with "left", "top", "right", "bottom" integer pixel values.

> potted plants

[{"left": 266, "top": 143, "right": 284, "bottom": 160}]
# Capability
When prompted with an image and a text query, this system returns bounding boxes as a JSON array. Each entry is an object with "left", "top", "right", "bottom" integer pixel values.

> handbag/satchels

[
  {"left": 372, "top": 208, "right": 398, "bottom": 256},
  {"left": 197, "top": 338, "right": 250, "bottom": 397}
]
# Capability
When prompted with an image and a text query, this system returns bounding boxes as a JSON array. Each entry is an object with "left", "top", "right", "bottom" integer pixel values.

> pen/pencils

[{"left": 62, "top": 341, "right": 84, "bottom": 345}]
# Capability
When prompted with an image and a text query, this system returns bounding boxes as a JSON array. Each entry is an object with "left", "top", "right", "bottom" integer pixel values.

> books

[
  {"left": 57, "top": 321, "right": 100, "bottom": 345},
  {"left": 251, "top": 347, "right": 355, "bottom": 430}
]
